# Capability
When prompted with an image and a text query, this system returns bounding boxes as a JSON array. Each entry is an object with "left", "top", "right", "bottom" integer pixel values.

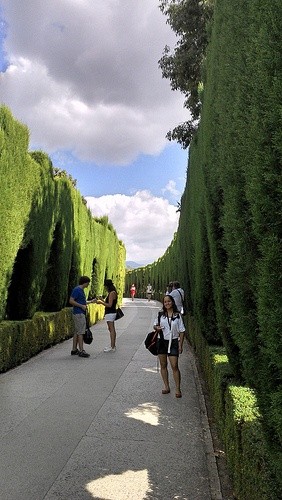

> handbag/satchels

[
  {"left": 115, "top": 307, "right": 124, "bottom": 321},
  {"left": 145, "top": 329, "right": 163, "bottom": 355},
  {"left": 84, "top": 329, "right": 93, "bottom": 344}
]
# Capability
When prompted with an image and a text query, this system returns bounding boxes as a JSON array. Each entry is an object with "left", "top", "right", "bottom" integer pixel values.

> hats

[{"left": 103, "top": 279, "right": 114, "bottom": 286}]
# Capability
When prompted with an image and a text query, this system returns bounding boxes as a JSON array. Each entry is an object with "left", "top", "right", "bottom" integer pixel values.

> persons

[
  {"left": 130, "top": 284, "right": 136, "bottom": 301},
  {"left": 166, "top": 281, "right": 185, "bottom": 314},
  {"left": 69, "top": 276, "right": 99, "bottom": 358},
  {"left": 96, "top": 279, "right": 118, "bottom": 353},
  {"left": 153, "top": 294, "right": 186, "bottom": 398},
  {"left": 146, "top": 283, "right": 153, "bottom": 302}
]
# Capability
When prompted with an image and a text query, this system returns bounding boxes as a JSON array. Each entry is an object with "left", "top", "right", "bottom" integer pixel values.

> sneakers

[
  {"left": 79, "top": 350, "right": 89, "bottom": 357},
  {"left": 71, "top": 349, "right": 80, "bottom": 355}
]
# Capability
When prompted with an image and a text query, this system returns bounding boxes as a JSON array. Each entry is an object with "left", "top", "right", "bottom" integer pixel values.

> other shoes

[
  {"left": 162, "top": 389, "right": 169, "bottom": 394},
  {"left": 175, "top": 393, "right": 182, "bottom": 397},
  {"left": 104, "top": 346, "right": 115, "bottom": 352}
]
